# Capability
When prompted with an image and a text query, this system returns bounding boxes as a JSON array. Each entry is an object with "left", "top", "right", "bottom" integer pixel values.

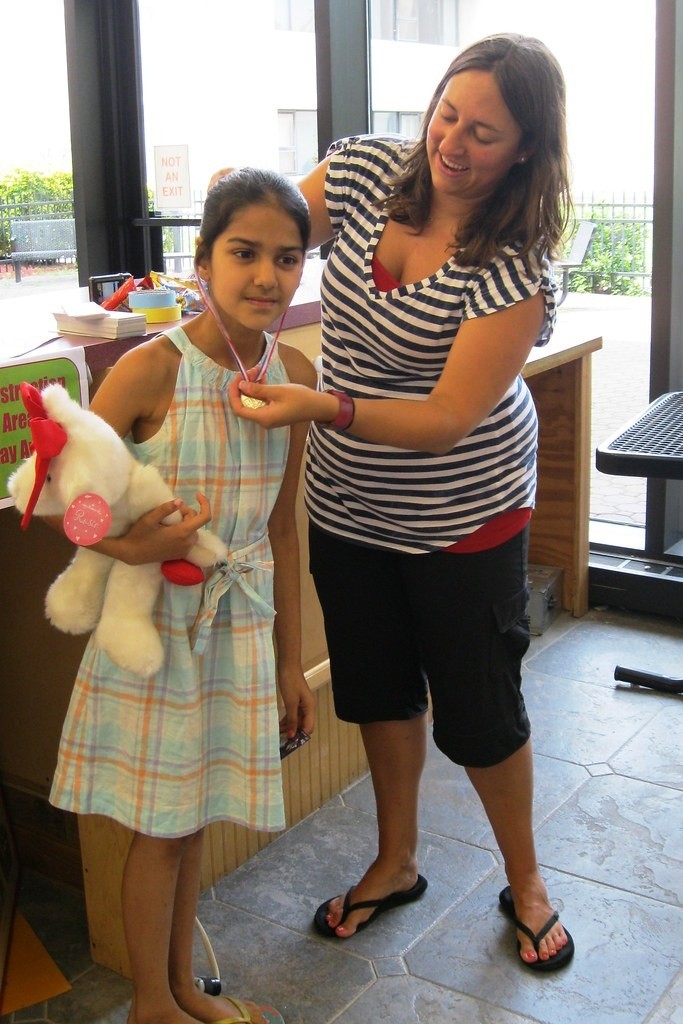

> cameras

[{"left": 89, "top": 272, "right": 132, "bottom": 305}]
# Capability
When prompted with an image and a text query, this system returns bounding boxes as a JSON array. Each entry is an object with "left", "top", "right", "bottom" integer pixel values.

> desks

[{"left": 522, "top": 333, "right": 598, "bottom": 620}]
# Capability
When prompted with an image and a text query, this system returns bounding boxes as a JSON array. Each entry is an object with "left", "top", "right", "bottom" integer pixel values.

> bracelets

[{"left": 313, "top": 385, "right": 356, "bottom": 435}]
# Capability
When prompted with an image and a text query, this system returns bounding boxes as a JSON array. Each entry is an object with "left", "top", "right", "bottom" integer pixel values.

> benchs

[{"left": 11, "top": 219, "right": 77, "bottom": 283}]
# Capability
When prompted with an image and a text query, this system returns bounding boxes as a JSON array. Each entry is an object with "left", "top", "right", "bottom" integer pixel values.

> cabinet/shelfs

[{"left": 0, "top": 435, "right": 370, "bottom": 906}]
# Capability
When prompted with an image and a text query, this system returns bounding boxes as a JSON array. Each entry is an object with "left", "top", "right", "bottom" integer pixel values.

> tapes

[
  {"left": 127, "top": 289, "right": 176, "bottom": 310},
  {"left": 132, "top": 304, "right": 182, "bottom": 324}
]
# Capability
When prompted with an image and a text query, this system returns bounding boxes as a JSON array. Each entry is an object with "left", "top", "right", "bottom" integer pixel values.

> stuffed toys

[{"left": 2, "top": 380, "right": 230, "bottom": 682}]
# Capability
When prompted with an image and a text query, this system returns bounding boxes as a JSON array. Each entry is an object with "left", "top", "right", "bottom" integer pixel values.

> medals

[{"left": 239, "top": 393, "right": 269, "bottom": 411}]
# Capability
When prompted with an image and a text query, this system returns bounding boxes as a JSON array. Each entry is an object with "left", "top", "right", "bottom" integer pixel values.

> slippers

[
  {"left": 313, "top": 873, "right": 429, "bottom": 939},
  {"left": 211, "top": 993, "right": 285, "bottom": 1024},
  {"left": 498, "top": 883, "right": 575, "bottom": 973}
]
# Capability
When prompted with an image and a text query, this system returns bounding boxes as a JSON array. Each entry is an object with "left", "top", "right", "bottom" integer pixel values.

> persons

[
  {"left": 203, "top": 32, "right": 578, "bottom": 976},
  {"left": 44, "top": 166, "right": 322, "bottom": 1023}
]
